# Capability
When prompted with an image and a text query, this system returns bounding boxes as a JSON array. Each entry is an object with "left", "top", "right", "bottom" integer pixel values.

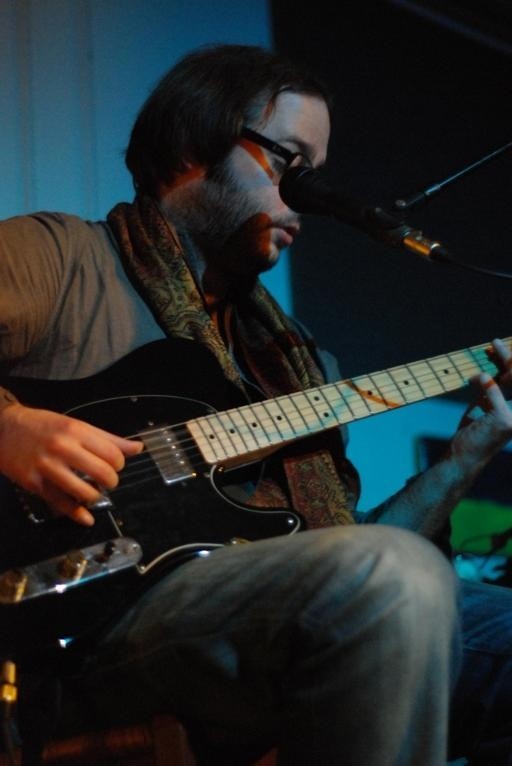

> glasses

[{"left": 243, "top": 127, "right": 313, "bottom": 170}]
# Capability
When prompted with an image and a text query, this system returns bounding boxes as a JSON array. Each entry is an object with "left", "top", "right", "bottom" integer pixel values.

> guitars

[{"left": 2, "top": 337, "right": 511, "bottom": 671}]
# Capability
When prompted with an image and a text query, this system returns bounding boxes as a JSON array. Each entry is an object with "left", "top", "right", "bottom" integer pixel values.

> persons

[{"left": 2, "top": 45, "right": 512, "bottom": 765}]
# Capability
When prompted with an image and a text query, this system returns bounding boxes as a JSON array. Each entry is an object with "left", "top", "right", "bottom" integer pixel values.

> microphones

[{"left": 279, "top": 166, "right": 443, "bottom": 262}]
